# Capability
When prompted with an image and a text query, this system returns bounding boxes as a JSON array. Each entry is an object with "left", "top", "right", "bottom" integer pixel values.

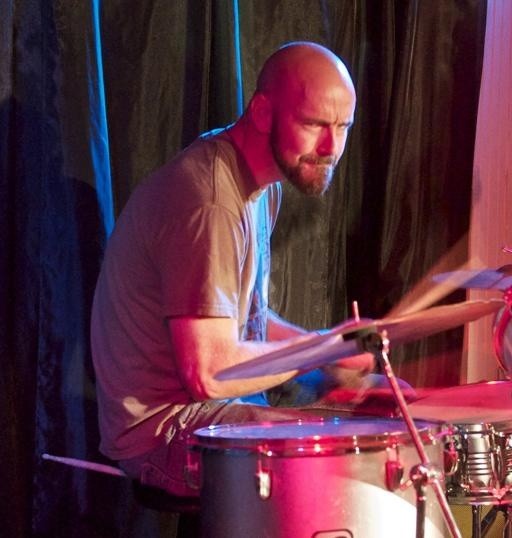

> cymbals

[
  {"left": 433, "top": 267, "right": 512, "bottom": 292},
  {"left": 213, "top": 300, "right": 505, "bottom": 382}
]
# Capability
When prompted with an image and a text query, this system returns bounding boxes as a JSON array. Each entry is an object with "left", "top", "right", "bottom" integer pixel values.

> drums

[
  {"left": 188, "top": 421, "right": 446, "bottom": 538},
  {"left": 444, "top": 382, "right": 512, "bottom": 506}
]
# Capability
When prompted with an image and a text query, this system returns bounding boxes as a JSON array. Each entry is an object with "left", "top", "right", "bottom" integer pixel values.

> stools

[{"left": 131, "top": 484, "right": 203, "bottom": 538}]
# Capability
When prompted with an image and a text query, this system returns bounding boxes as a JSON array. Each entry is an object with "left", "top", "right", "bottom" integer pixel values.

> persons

[{"left": 89, "top": 38, "right": 418, "bottom": 505}]
